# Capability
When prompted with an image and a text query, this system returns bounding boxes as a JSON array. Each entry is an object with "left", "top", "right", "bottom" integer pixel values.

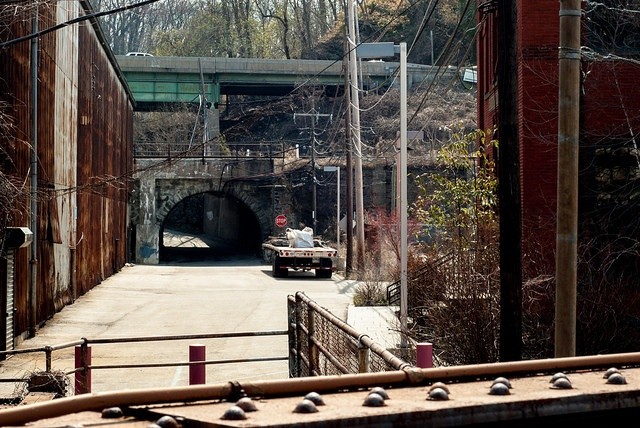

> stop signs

[{"left": 274, "top": 214, "right": 288, "bottom": 228}]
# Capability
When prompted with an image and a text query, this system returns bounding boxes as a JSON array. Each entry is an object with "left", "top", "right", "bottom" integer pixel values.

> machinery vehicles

[{"left": 264, "top": 231, "right": 336, "bottom": 279}]
